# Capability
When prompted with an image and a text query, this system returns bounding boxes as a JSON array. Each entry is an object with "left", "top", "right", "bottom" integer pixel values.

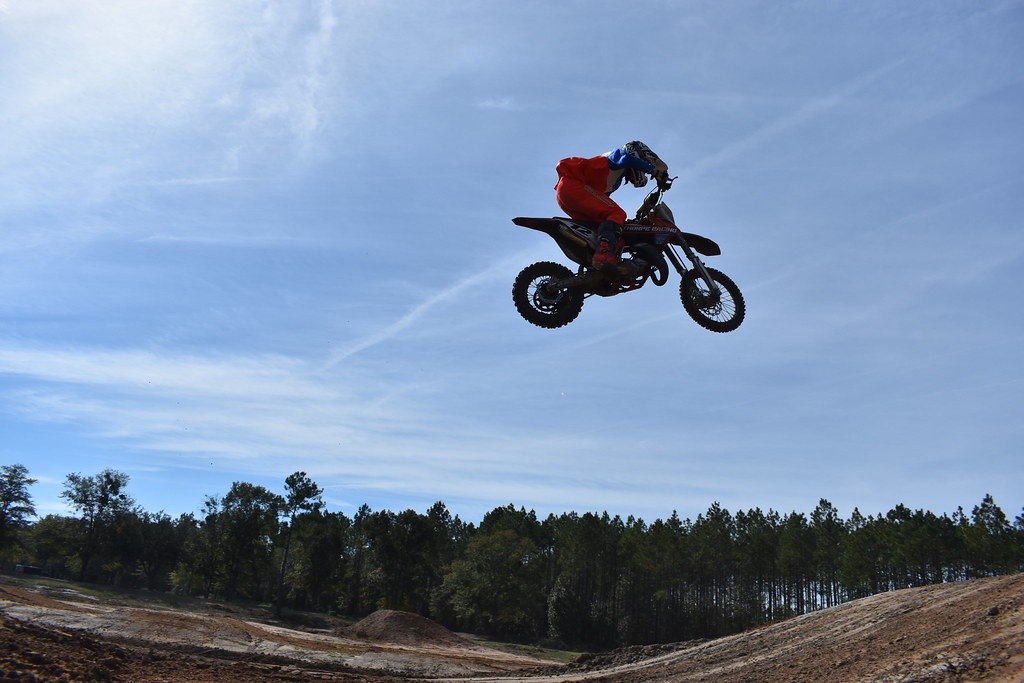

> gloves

[{"left": 650, "top": 166, "right": 669, "bottom": 183}]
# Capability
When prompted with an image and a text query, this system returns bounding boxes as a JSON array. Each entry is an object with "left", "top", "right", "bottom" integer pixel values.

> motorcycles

[{"left": 512, "top": 173, "right": 746, "bottom": 333}]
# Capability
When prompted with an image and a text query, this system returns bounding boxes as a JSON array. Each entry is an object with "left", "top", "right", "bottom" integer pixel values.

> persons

[{"left": 554, "top": 139, "right": 669, "bottom": 273}]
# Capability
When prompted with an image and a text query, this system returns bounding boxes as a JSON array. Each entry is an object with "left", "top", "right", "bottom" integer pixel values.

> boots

[{"left": 592, "top": 220, "right": 632, "bottom": 275}]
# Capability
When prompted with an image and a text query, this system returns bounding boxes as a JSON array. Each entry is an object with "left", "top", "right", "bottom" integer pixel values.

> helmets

[{"left": 621, "top": 140, "right": 668, "bottom": 187}]
